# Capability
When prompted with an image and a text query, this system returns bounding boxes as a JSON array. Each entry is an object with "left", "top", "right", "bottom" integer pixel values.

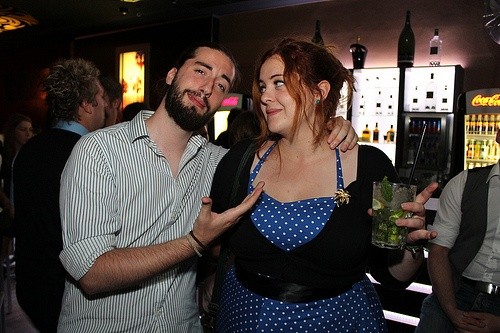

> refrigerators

[{"left": 455, "top": 87, "right": 500, "bottom": 174}]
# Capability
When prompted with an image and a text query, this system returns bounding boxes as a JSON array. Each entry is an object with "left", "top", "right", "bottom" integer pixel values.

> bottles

[
  {"left": 373, "top": 122, "right": 379, "bottom": 143},
  {"left": 467, "top": 139, "right": 500, "bottom": 160},
  {"left": 466, "top": 115, "right": 500, "bottom": 135},
  {"left": 387, "top": 125, "right": 395, "bottom": 143},
  {"left": 362, "top": 124, "right": 371, "bottom": 142}
]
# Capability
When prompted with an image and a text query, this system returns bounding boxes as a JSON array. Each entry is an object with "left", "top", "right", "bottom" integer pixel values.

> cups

[{"left": 371, "top": 181, "right": 417, "bottom": 250}]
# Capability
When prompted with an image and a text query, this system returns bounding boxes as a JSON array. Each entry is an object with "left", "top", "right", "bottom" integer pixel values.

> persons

[
  {"left": 203, "top": 38, "right": 439, "bottom": 333},
  {"left": 225, "top": 111, "right": 265, "bottom": 150},
  {"left": 58, "top": 40, "right": 359, "bottom": 333},
  {"left": 0, "top": 114, "right": 33, "bottom": 280},
  {"left": 11, "top": 59, "right": 152, "bottom": 333},
  {"left": 414, "top": 130, "right": 500, "bottom": 333}
]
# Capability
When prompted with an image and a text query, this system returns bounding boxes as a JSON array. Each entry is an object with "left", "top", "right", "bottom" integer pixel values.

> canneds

[{"left": 411, "top": 120, "right": 441, "bottom": 135}]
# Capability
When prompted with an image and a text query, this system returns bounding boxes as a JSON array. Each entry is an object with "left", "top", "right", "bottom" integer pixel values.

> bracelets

[{"left": 186, "top": 230, "right": 207, "bottom": 257}]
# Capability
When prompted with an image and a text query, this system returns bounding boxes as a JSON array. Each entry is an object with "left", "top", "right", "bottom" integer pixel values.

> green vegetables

[{"left": 371, "top": 176, "right": 416, "bottom": 245}]
